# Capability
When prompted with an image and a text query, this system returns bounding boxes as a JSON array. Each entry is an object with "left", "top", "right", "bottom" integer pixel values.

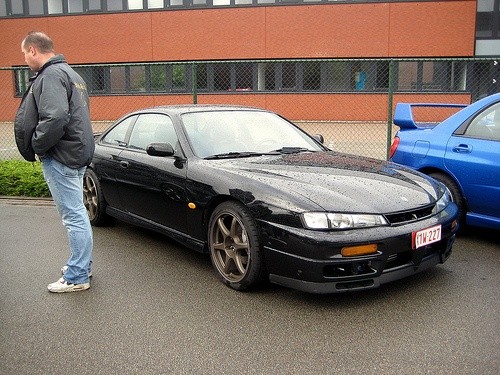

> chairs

[
  {"left": 470, "top": 124, "right": 493, "bottom": 137},
  {"left": 154, "top": 125, "right": 178, "bottom": 149}
]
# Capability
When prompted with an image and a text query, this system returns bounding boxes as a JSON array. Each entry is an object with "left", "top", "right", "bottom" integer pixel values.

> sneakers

[
  {"left": 61, "top": 265, "right": 92, "bottom": 276},
  {"left": 47, "top": 276, "right": 90, "bottom": 293}
]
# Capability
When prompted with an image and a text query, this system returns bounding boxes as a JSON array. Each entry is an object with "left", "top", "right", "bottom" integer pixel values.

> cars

[
  {"left": 81, "top": 103, "right": 461, "bottom": 297},
  {"left": 386, "top": 91, "right": 500, "bottom": 237}
]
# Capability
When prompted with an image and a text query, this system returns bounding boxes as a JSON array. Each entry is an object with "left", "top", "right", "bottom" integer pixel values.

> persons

[{"left": 13, "top": 32, "right": 96, "bottom": 293}]
